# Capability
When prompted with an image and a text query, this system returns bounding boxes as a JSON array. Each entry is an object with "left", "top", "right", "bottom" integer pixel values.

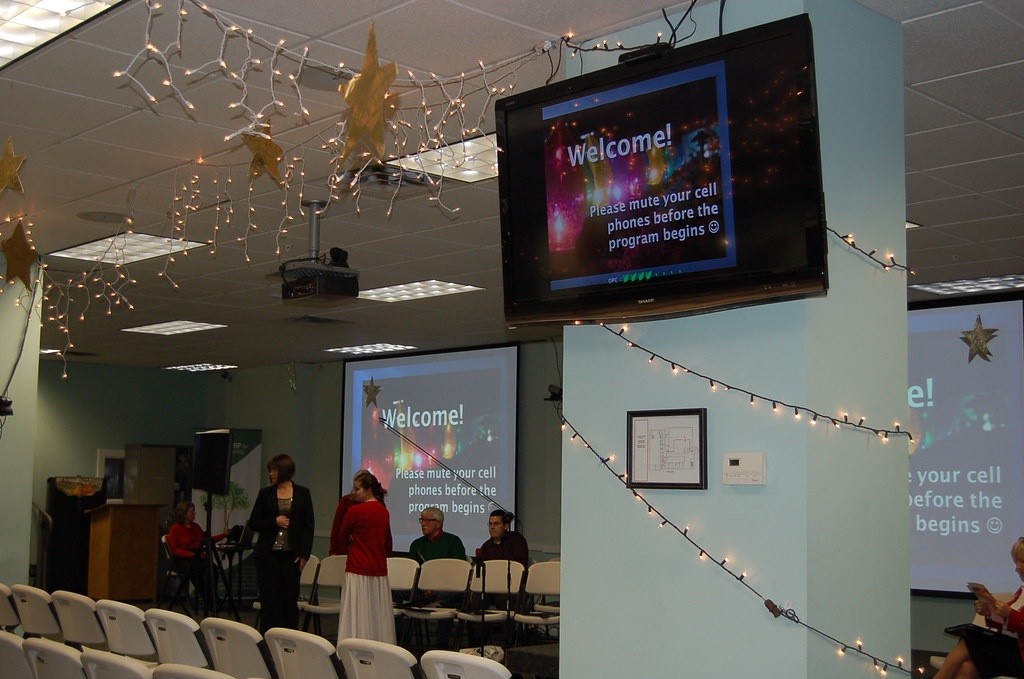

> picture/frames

[{"left": 625, "top": 409, "right": 706, "bottom": 491}]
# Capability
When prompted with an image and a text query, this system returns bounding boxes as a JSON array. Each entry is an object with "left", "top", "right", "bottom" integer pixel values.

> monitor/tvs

[{"left": 494, "top": 13, "right": 829, "bottom": 329}]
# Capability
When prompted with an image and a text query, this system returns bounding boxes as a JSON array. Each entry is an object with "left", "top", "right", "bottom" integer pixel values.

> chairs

[
  {"left": 929, "top": 593, "right": 1024, "bottom": 679},
  {"left": 0, "top": 526, "right": 561, "bottom": 679}
]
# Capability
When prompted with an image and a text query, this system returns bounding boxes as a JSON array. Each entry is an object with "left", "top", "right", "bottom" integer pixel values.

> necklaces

[{"left": 279, "top": 487, "right": 285, "bottom": 494}]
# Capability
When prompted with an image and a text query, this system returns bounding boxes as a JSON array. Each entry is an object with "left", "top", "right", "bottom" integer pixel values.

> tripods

[{"left": 167, "top": 492, "right": 242, "bottom": 626}]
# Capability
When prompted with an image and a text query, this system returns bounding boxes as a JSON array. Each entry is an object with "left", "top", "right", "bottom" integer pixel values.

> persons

[
  {"left": 475, "top": 509, "right": 530, "bottom": 642},
  {"left": 245, "top": 454, "right": 314, "bottom": 632},
  {"left": 927, "top": 536, "right": 1024, "bottom": 679},
  {"left": 331, "top": 468, "right": 397, "bottom": 651},
  {"left": 408, "top": 507, "right": 467, "bottom": 648},
  {"left": 164, "top": 501, "right": 231, "bottom": 614}
]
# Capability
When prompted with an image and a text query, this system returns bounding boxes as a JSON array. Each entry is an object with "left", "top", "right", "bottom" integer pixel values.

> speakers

[{"left": 191, "top": 433, "right": 233, "bottom": 495}]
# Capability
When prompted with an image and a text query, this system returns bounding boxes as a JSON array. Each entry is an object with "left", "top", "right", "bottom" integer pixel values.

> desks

[{"left": 201, "top": 542, "right": 256, "bottom": 601}]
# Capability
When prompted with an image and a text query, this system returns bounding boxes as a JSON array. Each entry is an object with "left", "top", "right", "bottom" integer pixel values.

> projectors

[{"left": 282, "top": 273, "right": 359, "bottom": 303}]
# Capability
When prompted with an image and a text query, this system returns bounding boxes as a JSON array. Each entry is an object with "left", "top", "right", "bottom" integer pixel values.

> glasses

[
  {"left": 487, "top": 521, "right": 502, "bottom": 527},
  {"left": 419, "top": 517, "right": 440, "bottom": 524}
]
